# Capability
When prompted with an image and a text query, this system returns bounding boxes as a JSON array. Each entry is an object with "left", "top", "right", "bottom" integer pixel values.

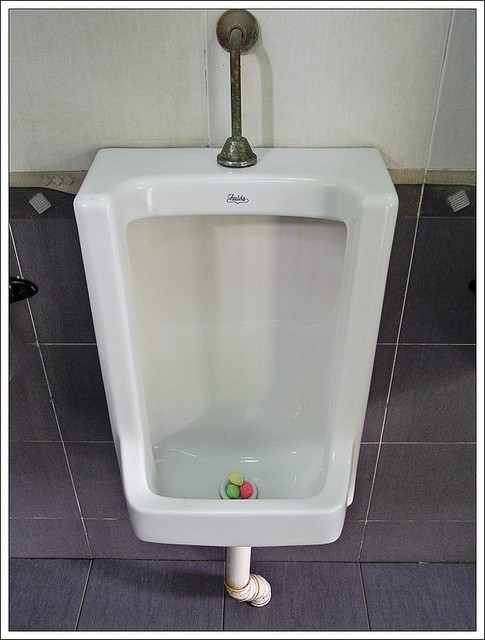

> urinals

[{"left": 72, "top": 8, "right": 401, "bottom": 546}]
